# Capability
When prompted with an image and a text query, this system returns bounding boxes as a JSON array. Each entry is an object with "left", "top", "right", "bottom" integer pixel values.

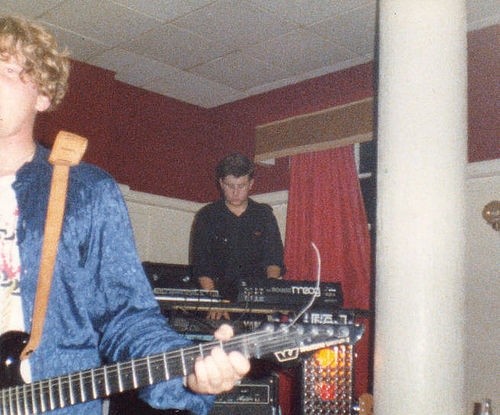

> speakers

[{"left": 207, "top": 374, "right": 279, "bottom": 415}]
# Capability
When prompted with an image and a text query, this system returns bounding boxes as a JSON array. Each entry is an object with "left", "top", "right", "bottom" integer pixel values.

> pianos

[{"left": 154, "top": 287, "right": 221, "bottom": 307}]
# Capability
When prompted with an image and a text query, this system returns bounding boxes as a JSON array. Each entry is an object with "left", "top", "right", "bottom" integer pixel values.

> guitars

[{"left": 0, "top": 321, "right": 368, "bottom": 415}]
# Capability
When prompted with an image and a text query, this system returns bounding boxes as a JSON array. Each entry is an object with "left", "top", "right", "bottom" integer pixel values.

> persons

[
  {"left": 1, "top": 16, "right": 249, "bottom": 415},
  {"left": 189, "top": 156, "right": 285, "bottom": 321}
]
